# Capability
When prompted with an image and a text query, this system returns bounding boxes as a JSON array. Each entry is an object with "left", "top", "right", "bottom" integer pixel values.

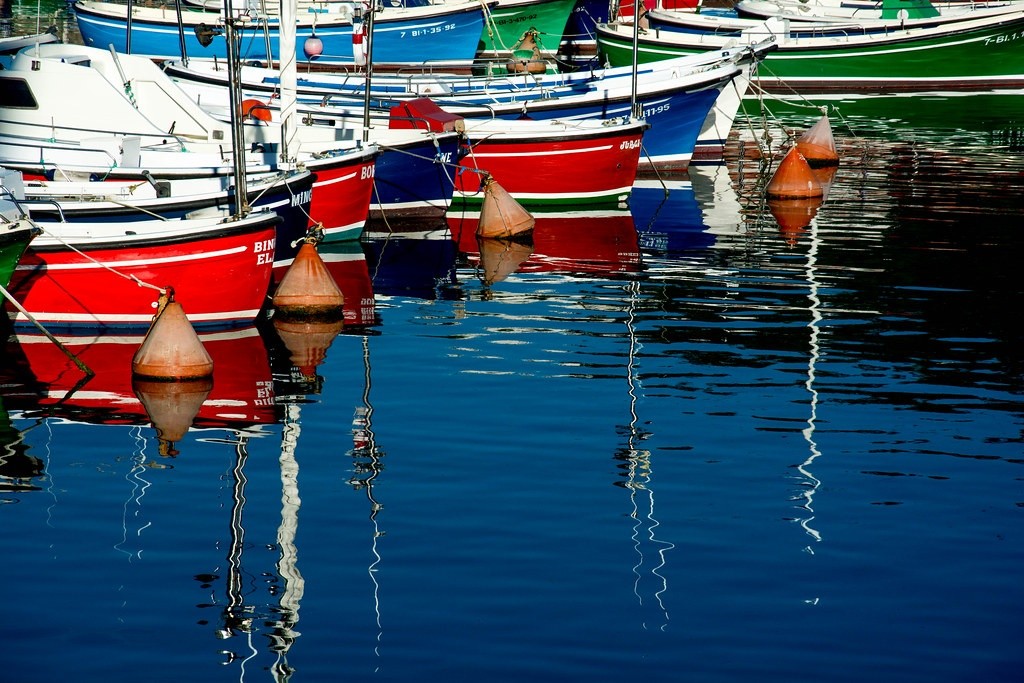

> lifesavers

[
  {"left": 240, "top": 99, "right": 273, "bottom": 122},
  {"left": 351, "top": 20, "right": 370, "bottom": 67}
]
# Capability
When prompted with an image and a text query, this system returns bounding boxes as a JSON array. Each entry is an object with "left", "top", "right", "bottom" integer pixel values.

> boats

[
  {"left": 257, "top": 239, "right": 383, "bottom": 683},
  {"left": 0, "top": 1, "right": 779, "bottom": 330},
  {"left": 688, "top": 151, "right": 750, "bottom": 237},
  {"left": 625, "top": 170, "right": 719, "bottom": 260},
  {"left": 3, "top": 323, "right": 286, "bottom": 683},
  {"left": 596, "top": 0, "right": 1024, "bottom": 90},
  {"left": 444, "top": 202, "right": 674, "bottom": 611},
  {"left": 361, "top": 219, "right": 461, "bottom": 300}
]
